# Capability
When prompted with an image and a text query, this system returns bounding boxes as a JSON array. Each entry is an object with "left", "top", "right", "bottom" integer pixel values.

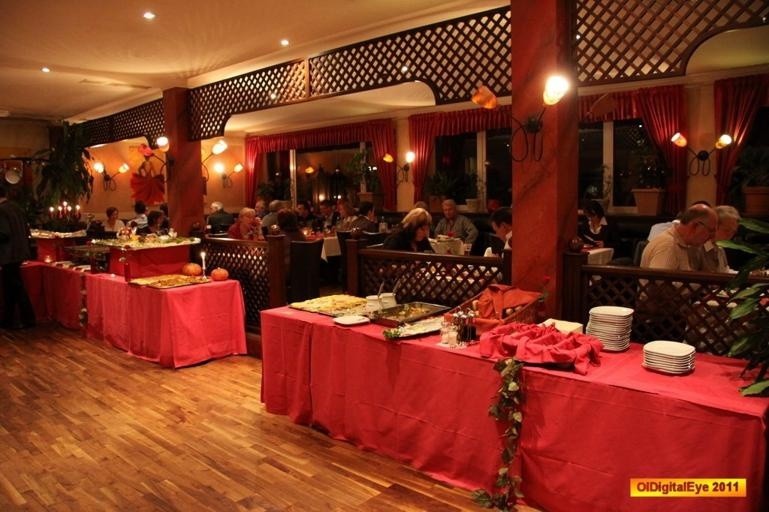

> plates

[
  {"left": 331, "top": 314, "right": 372, "bottom": 326},
  {"left": 584, "top": 305, "right": 634, "bottom": 353},
  {"left": 643, "top": 339, "right": 696, "bottom": 376}
]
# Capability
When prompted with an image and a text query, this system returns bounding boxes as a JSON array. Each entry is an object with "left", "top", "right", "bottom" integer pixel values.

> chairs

[{"left": 206, "top": 211, "right": 739, "bottom": 351}]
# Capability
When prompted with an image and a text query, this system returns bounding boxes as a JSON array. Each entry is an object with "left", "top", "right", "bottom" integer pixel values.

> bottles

[
  {"left": 365, "top": 292, "right": 397, "bottom": 315},
  {"left": 380, "top": 216, "right": 387, "bottom": 232},
  {"left": 441, "top": 310, "right": 476, "bottom": 349}
]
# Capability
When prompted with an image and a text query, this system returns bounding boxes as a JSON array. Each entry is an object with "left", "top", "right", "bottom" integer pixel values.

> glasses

[{"left": 700, "top": 221, "right": 715, "bottom": 234}]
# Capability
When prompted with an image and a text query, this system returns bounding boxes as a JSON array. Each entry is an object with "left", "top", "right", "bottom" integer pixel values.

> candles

[
  {"left": 48, "top": 201, "right": 82, "bottom": 221},
  {"left": 201, "top": 252, "right": 206, "bottom": 269}
]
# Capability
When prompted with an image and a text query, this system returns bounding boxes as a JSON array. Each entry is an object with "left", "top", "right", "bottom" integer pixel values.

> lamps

[
  {"left": 669, "top": 128, "right": 731, "bottom": 179},
  {"left": 214, "top": 163, "right": 243, "bottom": 190},
  {"left": 5, "top": 159, "right": 23, "bottom": 184},
  {"left": 472, "top": 75, "right": 571, "bottom": 162},
  {"left": 201, "top": 139, "right": 230, "bottom": 169},
  {"left": 93, "top": 160, "right": 130, "bottom": 192},
  {"left": 138, "top": 136, "right": 177, "bottom": 183},
  {"left": 382, "top": 151, "right": 415, "bottom": 186}
]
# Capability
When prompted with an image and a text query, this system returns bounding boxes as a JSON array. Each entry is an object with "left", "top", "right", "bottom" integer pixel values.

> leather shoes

[{"left": 10, "top": 320, "right": 36, "bottom": 331}]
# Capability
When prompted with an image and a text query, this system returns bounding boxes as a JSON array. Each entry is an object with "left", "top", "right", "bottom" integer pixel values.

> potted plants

[
  {"left": 584, "top": 161, "right": 614, "bottom": 216},
  {"left": 422, "top": 170, "right": 448, "bottom": 212},
  {"left": 466, "top": 168, "right": 480, "bottom": 211},
  {"left": 729, "top": 146, "right": 769, "bottom": 216},
  {"left": 346, "top": 148, "right": 386, "bottom": 211},
  {"left": 628, "top": 147, "right": 667, "bottom": 218}
]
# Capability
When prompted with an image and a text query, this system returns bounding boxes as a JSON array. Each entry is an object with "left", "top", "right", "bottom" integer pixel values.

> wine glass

[{"left": 423, "top": 250, "right": 433, "bottom": 269}]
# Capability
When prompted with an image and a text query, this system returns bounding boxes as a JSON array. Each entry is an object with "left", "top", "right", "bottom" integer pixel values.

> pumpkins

[
  {"left": 182, "top": 263, "right": 202, "bottom": 276},
  {"left": 211, "top": 267, "right": 229, "bottom": 280}
]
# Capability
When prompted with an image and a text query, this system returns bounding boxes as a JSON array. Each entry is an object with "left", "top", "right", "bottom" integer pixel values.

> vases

[{"left": 488, "top": 199, "right": 502, "bottom": 212}]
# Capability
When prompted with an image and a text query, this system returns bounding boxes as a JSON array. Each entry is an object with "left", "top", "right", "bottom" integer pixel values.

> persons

[
  {"left": 0, "top": 187, "right": 36, "bottom": 330},
  {"left": 637, "top": 200, "right": 740, "bottom": 343},
  {"left": 100, "top": 200, "right": 169, "bottom": 236},
  {"left": 578, "top": 201, "right": 623, "bottom": 258}
]
